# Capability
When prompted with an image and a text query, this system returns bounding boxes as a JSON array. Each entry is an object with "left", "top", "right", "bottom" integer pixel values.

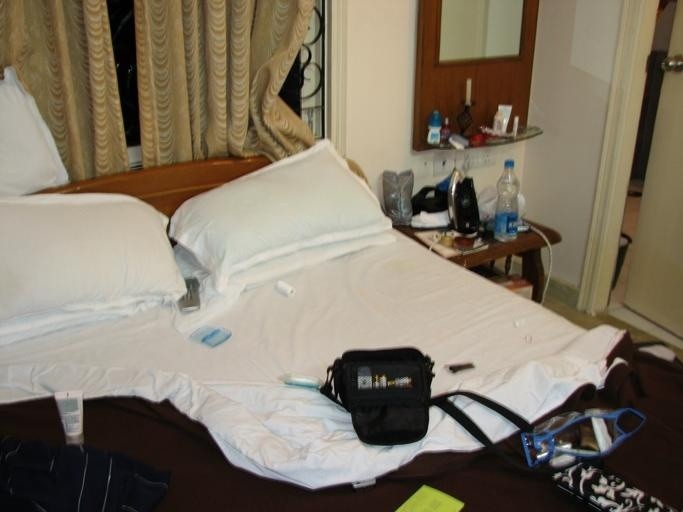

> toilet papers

[{"left": 274, "top": 280, "right": 295, "bottom": 297}]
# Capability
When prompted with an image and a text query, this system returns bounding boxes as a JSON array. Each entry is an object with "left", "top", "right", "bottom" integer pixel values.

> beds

[{"left": 0, "top": 154, "right": 683, "bottom": 512}]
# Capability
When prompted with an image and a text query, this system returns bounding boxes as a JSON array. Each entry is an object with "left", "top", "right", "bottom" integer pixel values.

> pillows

[
  {"left": 0, "top": 66, "right": 70, "bottom": 200},
  {"left": 167, "top": 136, "right": 396, "bottom": 291},
  {"left": 0, "top": 194, "right": 190, "bottom": 347}
]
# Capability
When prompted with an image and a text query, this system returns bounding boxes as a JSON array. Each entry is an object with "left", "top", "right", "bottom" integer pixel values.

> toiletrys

[
  {"left": 492, "top": 111, "right": 504, "bottom": 136},
  {"left": 497, "top": 104, "right": 513, "bottom": 136}
]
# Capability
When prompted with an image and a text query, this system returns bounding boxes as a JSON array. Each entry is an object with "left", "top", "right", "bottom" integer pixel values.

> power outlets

[{"left": 476, "top": 150, "right": 495, "bottom": 167}]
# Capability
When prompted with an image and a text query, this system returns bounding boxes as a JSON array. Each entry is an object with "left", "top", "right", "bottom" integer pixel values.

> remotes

[{"left": 179, "top": 276, "right": 200, "bottom": 313}]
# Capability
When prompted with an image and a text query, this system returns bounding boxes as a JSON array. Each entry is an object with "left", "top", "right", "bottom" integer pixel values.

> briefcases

[{"left": 548, "top": 460, "right": 679, "bottom": 512}]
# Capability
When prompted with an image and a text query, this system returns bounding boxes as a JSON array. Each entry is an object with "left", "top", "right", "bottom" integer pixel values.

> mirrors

[{"left": 412, "top": 1, "right": 539, "bottom": 152}]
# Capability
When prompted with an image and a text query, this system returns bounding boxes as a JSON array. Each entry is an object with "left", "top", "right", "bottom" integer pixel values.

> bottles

[
  {"left": 494, "top": 160, "right": 520, "bottom": 242},
  {"left": 440, "top": 117, "right": 451, "bottom": 148}
]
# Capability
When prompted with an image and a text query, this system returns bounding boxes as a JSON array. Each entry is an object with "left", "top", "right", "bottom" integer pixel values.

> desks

[{"left": 390, "top": 216, "right": 562, "bottom": 301}]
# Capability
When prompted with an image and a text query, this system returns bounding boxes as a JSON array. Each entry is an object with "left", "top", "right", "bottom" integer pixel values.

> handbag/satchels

[
  {"left": 320, "top": 346, "right": 436, "bottom": 446},
  {"left": 0, "top": 435, "right": 171, "bottom": 512},
  {"left": 521, "top": 407, "right": 646, "bottom": 471}
]
